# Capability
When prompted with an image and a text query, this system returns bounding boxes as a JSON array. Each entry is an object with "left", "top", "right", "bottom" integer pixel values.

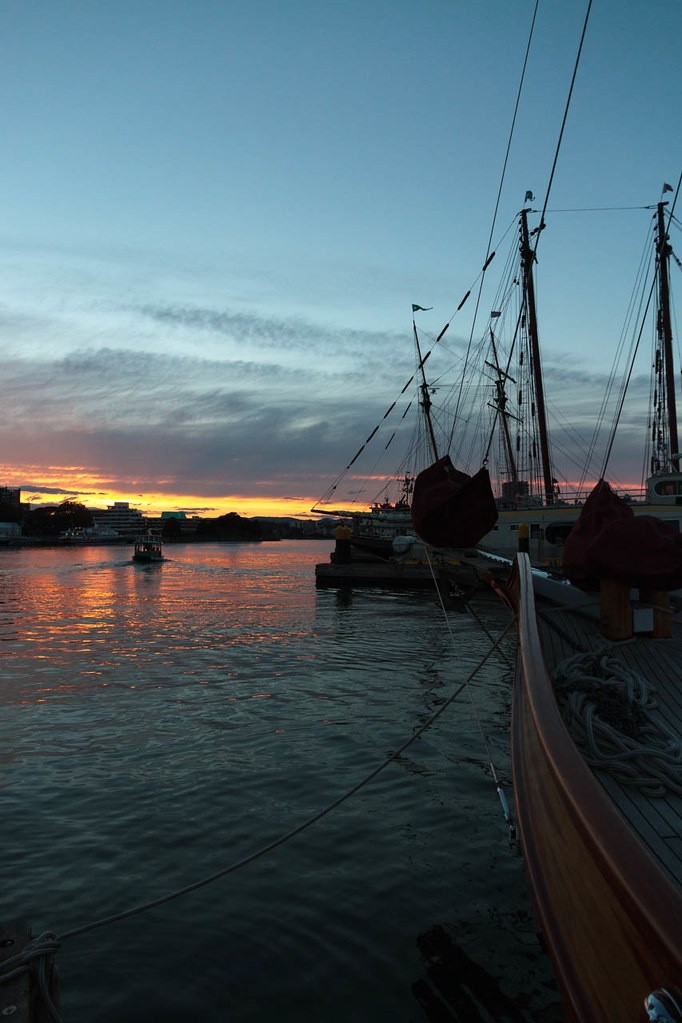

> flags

[
  {"left": 491, "top": 312, "right": 501, "bottom": 318},
  {"left": 524, "top": 190, "right": 535, "bottom": 203},
  {"left": 663, "top": 183, "right": 674, "bottom": 194},
  {"left": 413, "top": 304, "right": 433, "bottom": 312}
]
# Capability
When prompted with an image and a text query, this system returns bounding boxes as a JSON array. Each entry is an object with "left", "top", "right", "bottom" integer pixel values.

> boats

[{"left": 132, "top": 532, "right": 169, "bottom": 563}]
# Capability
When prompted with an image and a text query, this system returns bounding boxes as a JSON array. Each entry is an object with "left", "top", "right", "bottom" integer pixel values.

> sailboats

[{"left": 304, "top": 2, "right": 682, "bottom": 1023}]
